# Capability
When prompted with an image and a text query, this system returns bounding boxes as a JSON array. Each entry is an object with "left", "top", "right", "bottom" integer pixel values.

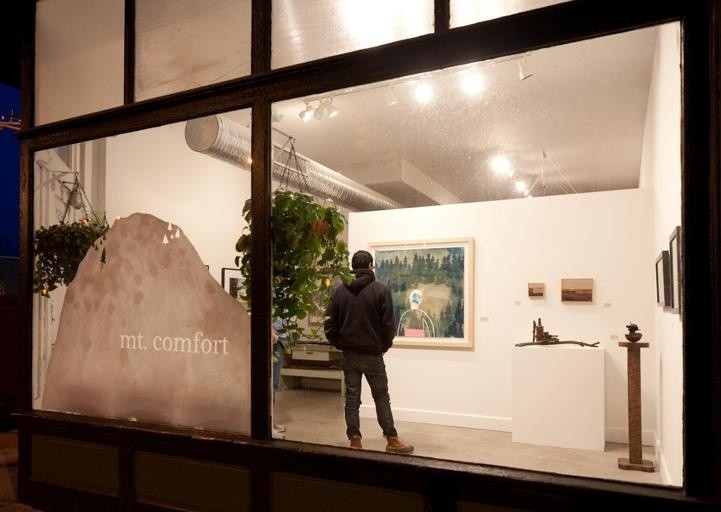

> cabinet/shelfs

[{"left": 272, "top": 338, "right": 347, "bottom": 396}]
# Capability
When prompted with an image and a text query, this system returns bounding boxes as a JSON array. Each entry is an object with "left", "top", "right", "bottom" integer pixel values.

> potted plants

[
  {"left": 226, "top": 188, "right": 355, "bottom": 363},
  {"left": 29, "top": 216, "right": 115, "bottom": 299}
]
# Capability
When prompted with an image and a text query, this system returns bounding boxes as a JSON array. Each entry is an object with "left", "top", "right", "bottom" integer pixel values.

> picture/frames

[
  {"left": 655, "top": 248, "right": 669, "bottom": 309},
  {"left": 668, "top": 223, "right": 682, "bottom": 314},
  {"left": 364, "top": 236, "right": 478, "bottom": 353},
  {"left": 221, "top": 266, "right": 250, "bottom": 315}
]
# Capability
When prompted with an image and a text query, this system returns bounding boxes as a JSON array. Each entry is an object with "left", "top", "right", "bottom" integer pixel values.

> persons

[
  {"left": 271, "top": 286, "right": 286, "bottom": 439},
  {"left": 324, "top": 251, "right": 414, "bottom": 454}
]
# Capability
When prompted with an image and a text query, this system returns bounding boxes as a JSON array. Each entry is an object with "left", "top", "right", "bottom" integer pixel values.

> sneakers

[
  {"left": 347, "top": 436, "right": 366, "bottom": 449},
  {"left": 385, "top": 438, "right": 414, "bottom": 453},
  {"left": 272, "top": 423, "right": 288, "bottom": 439}
]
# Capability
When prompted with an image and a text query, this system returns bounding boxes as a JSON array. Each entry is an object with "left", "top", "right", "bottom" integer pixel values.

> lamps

[{"left": 299, "top": 52, "right": 538, "bottom": 123}]
